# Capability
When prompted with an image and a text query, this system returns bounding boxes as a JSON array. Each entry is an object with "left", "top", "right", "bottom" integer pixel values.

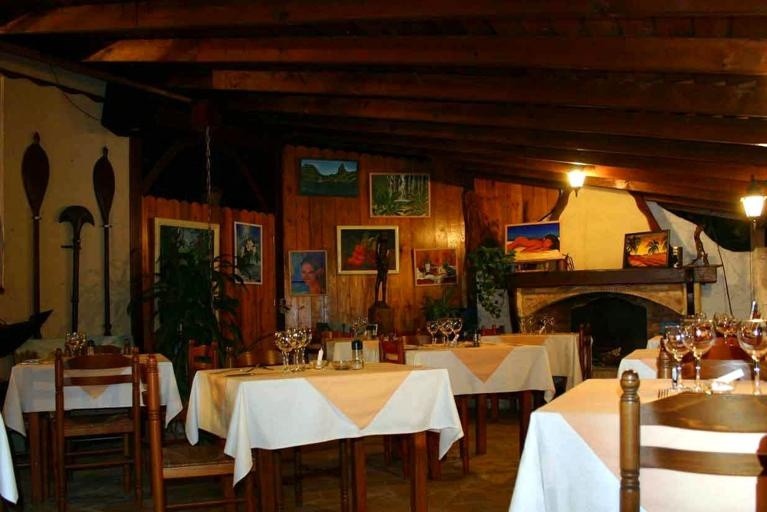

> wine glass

[
  {"left": 426, "top": 317, "right": 462, "bottom": 349},
  {"left": 352, "top": 315, "right": 369, "bottom": 340},
  {"left": 662, "top": 310, "right": 767, "bottom": 397},
  {"left": 274, "top": 326, "right": 312, "bottom": 374},
  {"left": 65, "top": 331, "right": 86, "bottom": 358}
]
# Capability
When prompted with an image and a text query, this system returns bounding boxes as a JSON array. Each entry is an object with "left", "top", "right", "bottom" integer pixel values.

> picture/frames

[
  {"left": 299, "top": 157, "right": 432, "bottom": 220},
  {"left": 151, "top": 216, "right": 221, "bottom": 338},
  {"left": 337, "top": 225, "right": 459, "bottom": 286},
  {"left": 234, "top": 221, "right": 329, "bottom": 299},
  {"left": 624, "top": 229, "right": 671, "bottom": 269},
  {"left": 503, "top": 221, "right": 563, "bottom": 261}
]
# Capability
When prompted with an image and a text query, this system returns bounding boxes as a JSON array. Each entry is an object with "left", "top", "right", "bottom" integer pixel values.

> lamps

[{"left": 740, "top": 172, "right": 767, "bottom": 224}]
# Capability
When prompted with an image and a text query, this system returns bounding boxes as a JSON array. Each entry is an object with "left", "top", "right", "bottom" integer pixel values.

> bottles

[
  {"left": 123, "top": 337, "right": 130, "bottom": 354},
  {"left": 471, "top": 324, "right": 481, "bottom": 347},
  {"left": 86, "top": 340, "right": 95, "bottom": 356},
  {"left": 351, "top": 340, "right": 364, "bottom": 371}
]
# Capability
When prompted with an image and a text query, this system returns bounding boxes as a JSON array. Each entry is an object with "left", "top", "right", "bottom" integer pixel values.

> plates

[{"left": 330, "top": 360, "right": 351, "bottom": 370}]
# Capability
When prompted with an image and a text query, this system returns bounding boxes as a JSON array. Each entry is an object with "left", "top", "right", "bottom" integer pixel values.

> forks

[{"left": 208, "top": 363, "right": 261, "bottom": 377}]
